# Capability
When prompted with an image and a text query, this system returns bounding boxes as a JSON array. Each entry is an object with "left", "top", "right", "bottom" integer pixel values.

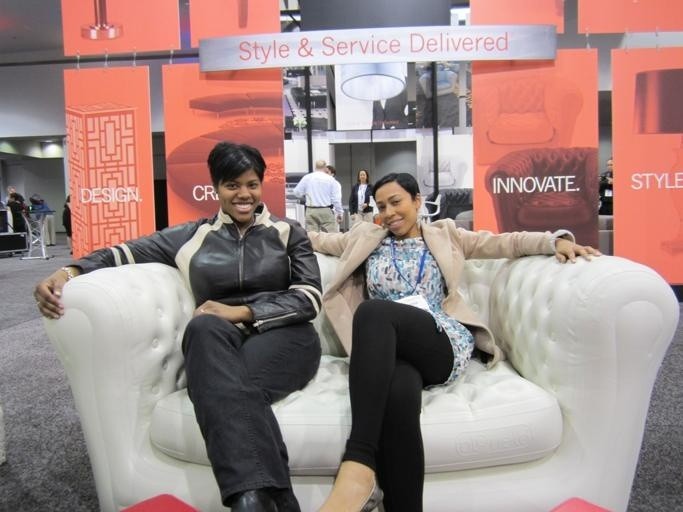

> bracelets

[{"left": 59, "top": 266, "right": 72, "bottom": 280}]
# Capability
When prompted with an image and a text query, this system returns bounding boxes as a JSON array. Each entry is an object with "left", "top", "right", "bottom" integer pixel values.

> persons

[
  {"left": 31, "top": 136, "right": 327, "bottom": 512},
  {"left": 326, "top": 164, "right": 337, "bottom": 178},
  {"left": 282, "top": 73, "right": 304, "bottom": 140},
  {"left": 598, "top": 155, "right": 614, "bottom": 219},
  {"left": 300, "top": 169, "right": 606, "bottom": 512},
  {"left": 61, "top": 193, "right": 74, "bottom": 256},
  {"left": 5, "top": 186, "right": 25, "bottom": 210},
  {"left": 292, "top": 159, "right": 345, "bottom": 235},
  {"left": 347, "top": 169, "right": 376, "bottom": 216},
  {"left": 29, "top": 192, "right": 57, "bottom": 247},
  {"left": 6, "top": 193, "right": 27, "bottom": 257},
  {"left": 418, "top": 62, "right": 462, "bottom": 126}
]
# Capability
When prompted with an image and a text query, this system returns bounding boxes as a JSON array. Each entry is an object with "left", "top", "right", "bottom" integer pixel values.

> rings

[
  {"left": 200, "top": 309, "right": 205, "bottom": 314},
  {"left": 35, "top": 301, "right": 41, "bottom": 306}
]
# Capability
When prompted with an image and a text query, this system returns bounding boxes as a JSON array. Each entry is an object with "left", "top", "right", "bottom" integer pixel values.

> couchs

[
  {"left": 40, "top": 244, "right": 680, "bottom": 512},
  {"left": 455, "top": 210, "right": 613, "bottom": 257}
]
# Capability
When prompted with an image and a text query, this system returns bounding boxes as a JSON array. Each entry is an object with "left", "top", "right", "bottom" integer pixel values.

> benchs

[{"left": 166, "top": 92, "right": 285, "bottom": 218}]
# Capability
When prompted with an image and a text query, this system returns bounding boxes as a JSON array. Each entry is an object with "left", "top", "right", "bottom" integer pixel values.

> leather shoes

[
  {"left": 358, "top": 472, "right": 385, "bottom": 512},
  {"left": 229, "top": 487, "right": 280, "bottom": 512}
]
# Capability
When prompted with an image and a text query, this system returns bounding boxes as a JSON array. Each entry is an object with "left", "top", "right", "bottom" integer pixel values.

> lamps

[
  {"left": 340, "top": 62, "right": 406, "bottom": 101},
  {"left": 632, "top": 68, "right": 683, "bottom": 252}
]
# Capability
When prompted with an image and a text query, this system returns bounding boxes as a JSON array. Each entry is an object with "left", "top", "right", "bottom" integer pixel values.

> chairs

[
  {"left": 484, "top": 145, "right": 598, "bottom": 248},
  {"left": 473, "top": 73, "right": 584, "bottom": 166}
]
